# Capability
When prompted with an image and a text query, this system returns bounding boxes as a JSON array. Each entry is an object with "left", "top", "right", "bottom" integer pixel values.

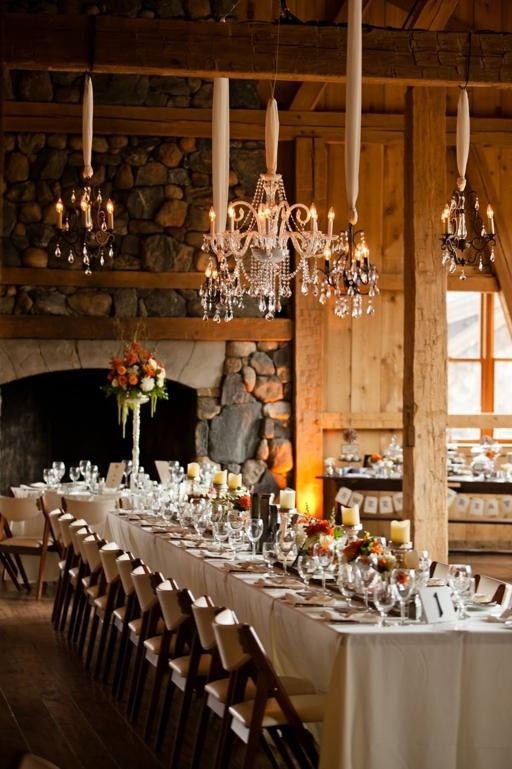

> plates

[
  {"left": 285, "top": 591, "right": 334, "bottom": 608},
  {"left": 261, "top": 576, "right": 306, "bottom": 590},
  {"left": 224, "top": 562, "right": 270, "bottom": 574},
  {"left": 328, "top": 612, "right": 382, "bottom": 624}
]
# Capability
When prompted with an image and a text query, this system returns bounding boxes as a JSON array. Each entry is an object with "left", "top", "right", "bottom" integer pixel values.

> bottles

[{"left": 418, "top": 550, "right": 431, "bottom": 584}]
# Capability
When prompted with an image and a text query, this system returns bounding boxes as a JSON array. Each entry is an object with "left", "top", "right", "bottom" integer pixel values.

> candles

[
  {"left": 187, "top": 463, "right": 199, "bottom": 476},
  {"left": 213, "top": 469, "right": 228, "bottom": 484},
  {"left": 340, "top": 504, "right": 360, "bottom": 525},
  {"left": 228, "top": 473, "right": 242, "bottom": 488},
  {"left": 280, "top": 488, "right": 295, "bottom": 508},
  {"left": 390, "top": 519, "right": 410, "bottom": 544}
]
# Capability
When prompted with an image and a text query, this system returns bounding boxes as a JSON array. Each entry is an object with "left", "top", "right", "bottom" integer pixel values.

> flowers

[{"left": 101, "top": 316, "right": 171, "bottom": 439}]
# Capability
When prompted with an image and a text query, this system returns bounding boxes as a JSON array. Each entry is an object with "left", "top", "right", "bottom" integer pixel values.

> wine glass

[
  {"left": 447, "top": 564, "right": 473, "bottom": 621},
  {"left": 190, "top": 497, "right": 264, "bottom": 560},
  {"left": 297, "top": 544, "right": 333, "bottom": 595},
  {"left": 42, "top": 460, "right": 105, "bottom": 494},
  {"left": 262, "top": 529, "right": 298, "bottom": 577},
  {"left": 336, "top": 553, "right": 416, "bottom": 628},
  {"left": 199, "top": 461, "right": 213, "bottom": 487},
  {"left": 121, "top": 459, "right": 190, "bottom": 528}
]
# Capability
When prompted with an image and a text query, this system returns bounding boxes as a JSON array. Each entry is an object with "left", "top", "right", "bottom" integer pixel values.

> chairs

[
  {"left": 0, "top": 494, "right": 62, "bottom": 600},
  {"left": 61, "top": 496, "right": 123, "bottom": 525},
  {"left": 47, "top": 506, "right": 326, "bottom": 769},
  {"left": 430, "top": 560, "right": 512, "bottom": 608}
]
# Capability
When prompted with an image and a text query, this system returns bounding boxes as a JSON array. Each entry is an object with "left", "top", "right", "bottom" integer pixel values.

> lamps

[
  {"left": 438, "top": 85, "right": 498, "bottom": 282},
  {"left": 52, "top": 69, "right": 117, "bottom": 277},
  {"left": 182, "top": 0, "right": 383, "bottom": 318}
]
